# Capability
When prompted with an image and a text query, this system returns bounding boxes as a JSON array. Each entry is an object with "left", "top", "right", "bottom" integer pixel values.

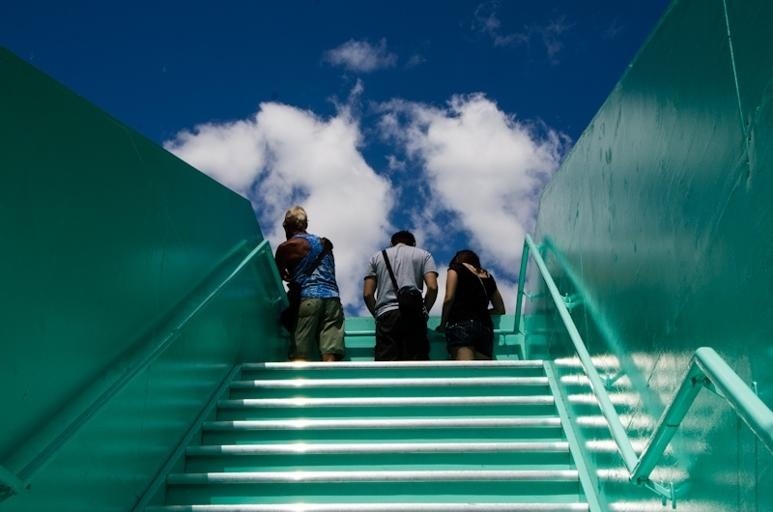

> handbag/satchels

[
  {"left": 398, "top": 285, "right": 423, "bottom": 320},
  {"left": 283, "top": 206, "right": 308, "bottom": 229},
  {"left": 468, "top": 315, "right": 494, "bottom": 355},
  {"left": 286, "top": 283, "right": 301, "bottom": 310}
]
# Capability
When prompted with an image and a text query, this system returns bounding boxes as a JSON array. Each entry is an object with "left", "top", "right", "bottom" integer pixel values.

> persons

[
  {"left": 276, "top": 207, "right": 347, "bottom": 361},
  {"left": 363, "top": 231, "right": 439, "bottom": 361},
  {"left": 435, "top": 249, "right": 506, "bottom": 361}
]
